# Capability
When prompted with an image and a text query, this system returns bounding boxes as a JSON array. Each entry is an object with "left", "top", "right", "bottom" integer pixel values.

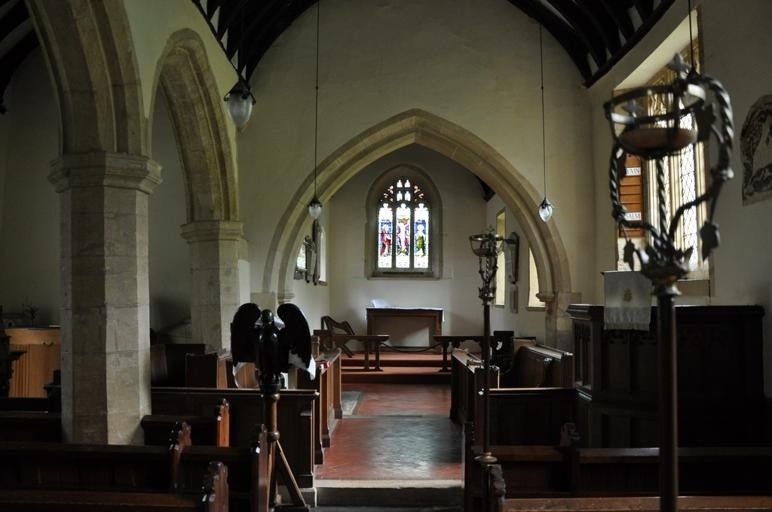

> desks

[{"left": 365, "top": 306, "right": 444, "bottom": 354}]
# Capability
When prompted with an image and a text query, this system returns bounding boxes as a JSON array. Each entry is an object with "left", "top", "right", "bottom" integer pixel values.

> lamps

[
  {"left": 308, "top": 5, "right": 322, "bottom": 220},
  {"left": 221, "top": 82, "right": 256, "bottom": 134},
  {"left": 536, "top": 23, "right": 554, "bottom": 223}
]
